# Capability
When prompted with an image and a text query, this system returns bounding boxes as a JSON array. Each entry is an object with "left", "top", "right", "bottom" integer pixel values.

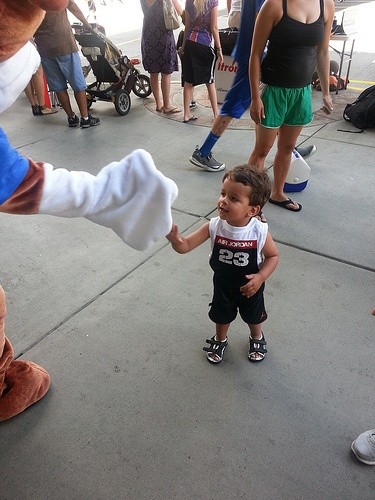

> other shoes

[{"left": 189, "top": 101, "right": 196, "bottom": 109}]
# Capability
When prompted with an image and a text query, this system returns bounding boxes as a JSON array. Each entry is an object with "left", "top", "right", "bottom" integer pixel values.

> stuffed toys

[{"left": 0, "top": 0, "right": 180, "bottom": 422}]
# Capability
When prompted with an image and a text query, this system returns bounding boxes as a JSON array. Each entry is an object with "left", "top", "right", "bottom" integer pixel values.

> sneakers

[
  {"left": 189, "top": 145, "right": 226, "bottom": 172},
  {"left": 68, "top": 112, "right": 80, "bottom": 127},
  {"left": 81, "top": 113, "right": 100, "bottom": 127},
  {"left": 350, "top": 428, "right": 374, "bottom": 465},
  {"left": 295, "top": 145, "right": 317, "bottom": 159}
]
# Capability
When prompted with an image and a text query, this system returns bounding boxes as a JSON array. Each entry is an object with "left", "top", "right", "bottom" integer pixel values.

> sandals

[
  {"left": 202, "top": 334, "right": 229, "bottom": 364},
  {"left": 247, "top": 330, "right": 267, "bottom": 362},
  {"left": 32, "top": 104, "right": 59, "bottom": 115}
]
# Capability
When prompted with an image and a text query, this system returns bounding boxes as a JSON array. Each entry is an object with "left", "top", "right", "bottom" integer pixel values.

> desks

[{"left": 329, "top": 34, "right": 357, "bottom": 94}]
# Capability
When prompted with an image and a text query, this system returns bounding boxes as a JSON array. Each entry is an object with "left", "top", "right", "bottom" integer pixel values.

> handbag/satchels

[
  {"left": 213, "top": 27, "right": 238, "bottom": 58},
  {"left": 163, "top": 0, "right": 181, "bottom": 30}
]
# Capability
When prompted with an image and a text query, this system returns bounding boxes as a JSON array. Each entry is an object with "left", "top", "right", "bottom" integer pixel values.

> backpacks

[
  {"left": 313, "top": 74, "right": 349, "bottom": 92},
  {"left": 342, "top": 84, "right": 374, "bottom": 129}
]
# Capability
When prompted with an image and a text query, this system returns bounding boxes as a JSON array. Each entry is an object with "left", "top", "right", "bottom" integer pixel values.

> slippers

[
  {"left": 183, "top": 114, "right": 198, "bottom": 122},
  {"left": 157, "top": 106, "right": 182, "bottom": 113},
  {"left": 269, "top": 197, "right": 302, "bottom": 212}
]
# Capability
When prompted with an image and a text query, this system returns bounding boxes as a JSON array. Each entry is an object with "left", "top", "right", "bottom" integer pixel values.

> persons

[
  {"left": 247, "top": 0, "right": 337, "bottom": 210},
  {"left": 176, "top": 0, "right": 318, "bottom": 171},
  {"left": 24, "top": 64, "right": 58, "bottom": 116},
  {"left": 140, "top": 0, "right": 184, "bottom": 113},
  {"left": 165, "top": 163, "right": 278, "bottom": 364},
  {"left": 34, "top": 0, "right": 100, "bottom": 128}
]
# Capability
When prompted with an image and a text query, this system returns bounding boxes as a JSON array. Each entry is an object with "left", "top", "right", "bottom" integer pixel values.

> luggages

[{"left": 214, "top": 55, "right": 239, "bottom": 104}]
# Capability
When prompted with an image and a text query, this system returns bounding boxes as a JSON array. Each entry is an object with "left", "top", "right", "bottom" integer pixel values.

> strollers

[{"left": 70, "top": 22, "right": 152, "bottom": 116}]
